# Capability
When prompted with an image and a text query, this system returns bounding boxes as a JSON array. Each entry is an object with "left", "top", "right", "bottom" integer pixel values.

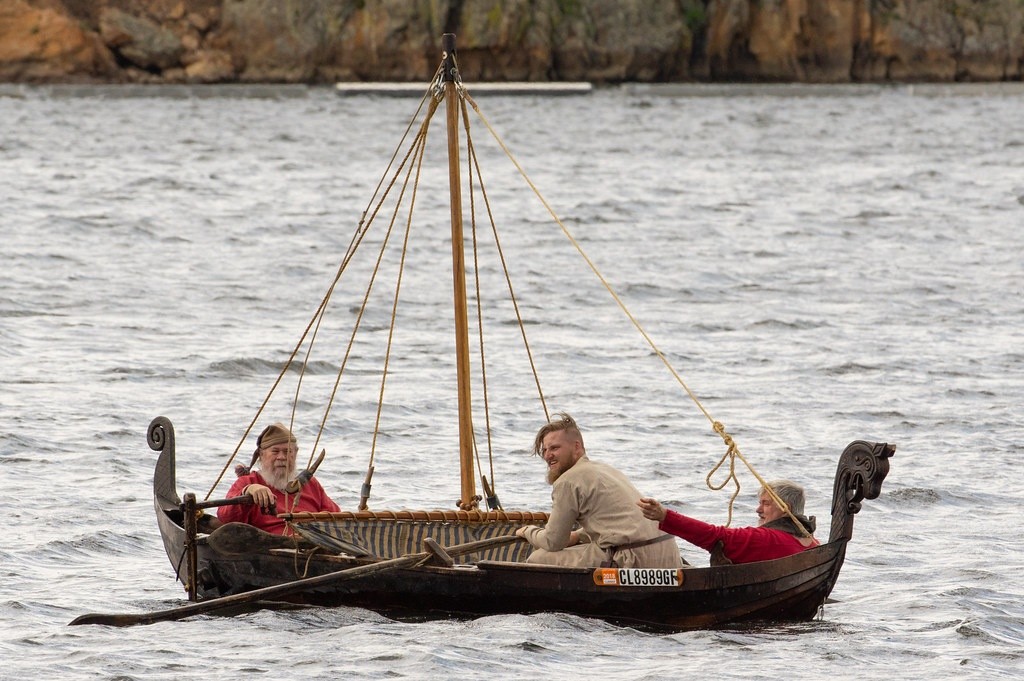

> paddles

[{"left": 67, "top": 532, "right": 526, "bottom": 630}]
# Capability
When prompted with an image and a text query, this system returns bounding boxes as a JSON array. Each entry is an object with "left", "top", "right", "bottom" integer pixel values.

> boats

[{"left": 147, "top": 34, "right": 896, "bottom": 633}]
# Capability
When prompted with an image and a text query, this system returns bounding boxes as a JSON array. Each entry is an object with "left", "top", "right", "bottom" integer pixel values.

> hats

[{"left": 235, "top": 422, "right": 296, "bottom": 477}]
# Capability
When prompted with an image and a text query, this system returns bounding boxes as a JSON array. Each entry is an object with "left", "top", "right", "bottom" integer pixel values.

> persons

[
  {"left": 636, "top": 479, "right": 820, "bottom": 564},
  {"left": 516, "top": 410, "right": 683, "bottom": 568},
  {"left": 217, "top": 422, "right": 341, "bottom": 536}
]
